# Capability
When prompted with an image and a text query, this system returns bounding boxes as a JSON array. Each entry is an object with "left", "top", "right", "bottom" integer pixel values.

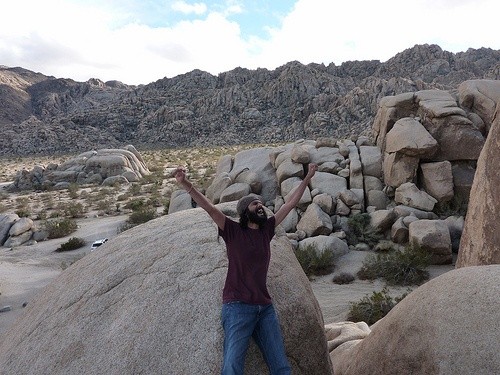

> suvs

[{"left": 90, "top": 238, "right": 109, "bottom": 252}]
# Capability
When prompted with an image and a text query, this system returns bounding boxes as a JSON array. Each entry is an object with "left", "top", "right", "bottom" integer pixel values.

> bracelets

[{"left": 188, "top": 185, "right": 193, "bottom": 193}]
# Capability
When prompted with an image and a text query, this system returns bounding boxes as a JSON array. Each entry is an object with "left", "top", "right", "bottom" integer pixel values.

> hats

[{"left": 237, "top": 194, "right": 260, "bottom": 217}]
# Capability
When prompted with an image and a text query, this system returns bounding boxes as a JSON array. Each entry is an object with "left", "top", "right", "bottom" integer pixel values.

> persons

[{"left": 175, "top": 165, "right": 318, "bottom": 375}]
